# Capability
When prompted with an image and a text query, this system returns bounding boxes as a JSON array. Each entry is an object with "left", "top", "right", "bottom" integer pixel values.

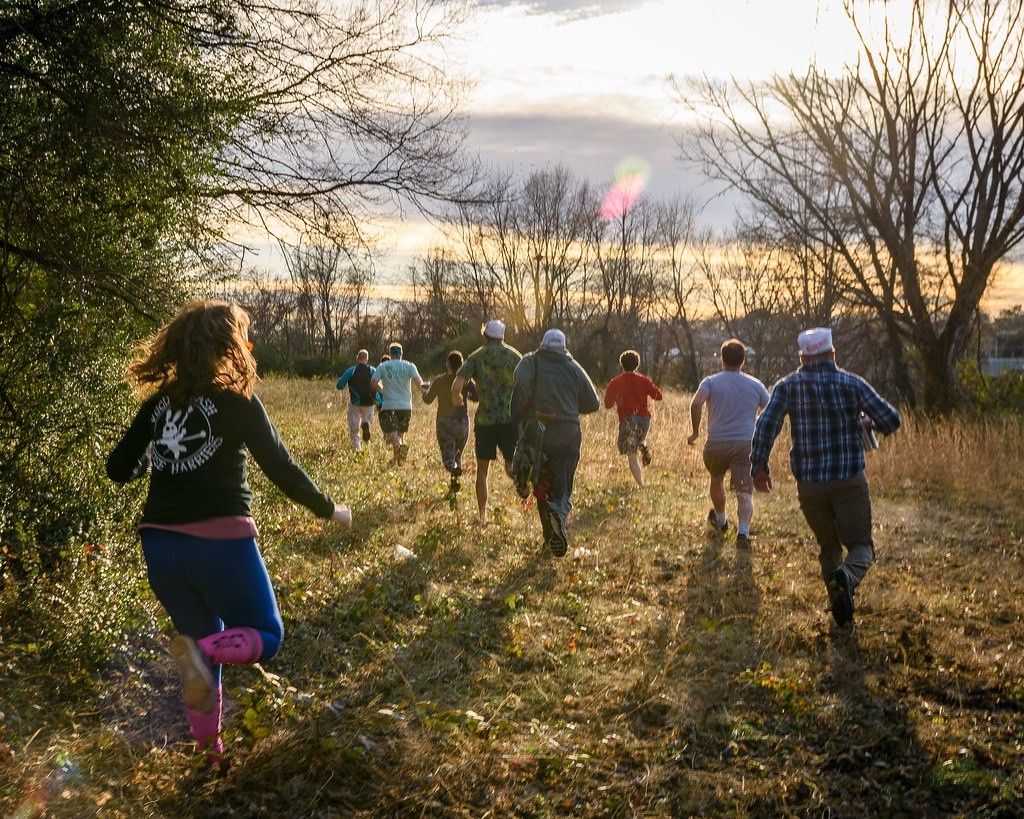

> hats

[
  {"left": 542, "top": 328, "right": 565, "bottom": 348},
  {"left": 485, "top": 319, "right": 506, "bottom": 339},
  {"left": 796, "top": 325, "right": 833, "bottom": 356}
]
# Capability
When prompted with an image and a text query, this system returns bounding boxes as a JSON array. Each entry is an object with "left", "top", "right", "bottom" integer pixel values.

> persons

[
  {"left": 510, "top": 327, "right": 600, "bottom": 559},
  {"left": 336, "top": 349, "right": 383, "bottom": 451},
  {"left": 749, "top": 327, "right": 900, "bottom": 627},
  {"left": 371, "top": 343, "right": 425, "bottom": 466},
  {"left": 604, "top": 350, "right": 662, "bottom": 490},
  {"left": 687, "top": 338, "right": 773, "bottom": 549},
  {"left": 421, "top": 351, "right": 479, "bottom": 494},
  {"left": 450, "top": 320, "right": 529, "bottom": 526},
  {"left": 104, "top": 300, "right": 352, "bottom": 773},
  {"left": 374, "top": 354, "right": 391, "bottom": 411}
]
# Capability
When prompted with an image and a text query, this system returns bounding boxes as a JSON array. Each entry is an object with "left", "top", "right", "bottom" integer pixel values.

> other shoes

[
  {"left": 514, "top": 454, "right": 532, "bottom": 498},
  {"left": 737, "top": 533, "right": 751, "bottom": 549},
  {"left": 451, "top": 467, "right": 462, "bottom": 493},
  {"left": 707, "top": 509, "right": 729, "bottom": 532},
  {"left": 639, "top": 444, "right": 652, "bottom": 466},
  {"left": 398, "top": 445, "right": 409, "bottom": 466},
  {"left": 361, "top": 421, "right": 371, "bottom": 442}
]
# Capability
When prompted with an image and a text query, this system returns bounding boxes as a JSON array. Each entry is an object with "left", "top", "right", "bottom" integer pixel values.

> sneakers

[
  {"left": 170, "top": 635, "right": 219, "bottom": 716},
  {"left": 829, "top": 567, "right": 856, "bottom": 629},
  {"left": 542, "top": 508, "right": 568, "bottom": 557},
  {"left": 196, "top": 758, "right": 242, "bottom": 783}
]
